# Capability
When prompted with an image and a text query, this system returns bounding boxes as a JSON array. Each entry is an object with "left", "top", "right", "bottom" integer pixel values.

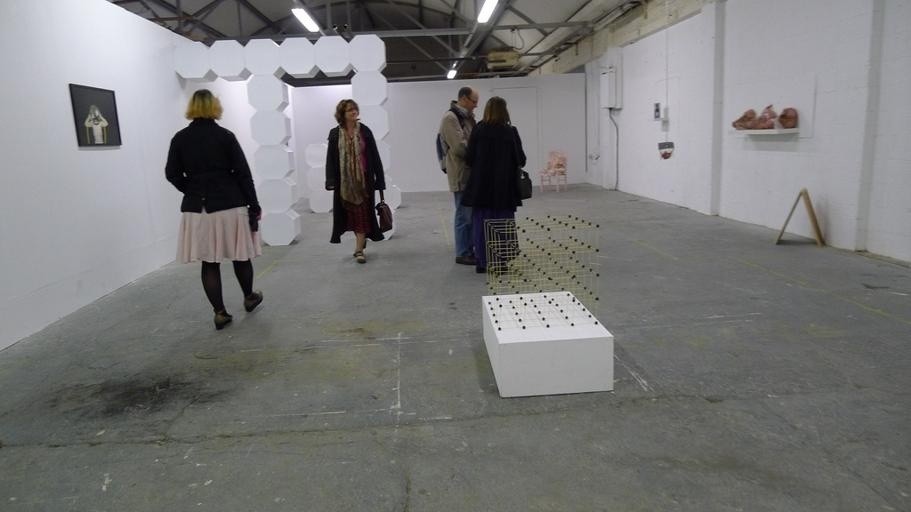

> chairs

[{"left": 540, "top": 149, "right": 570, "bottom": 194}]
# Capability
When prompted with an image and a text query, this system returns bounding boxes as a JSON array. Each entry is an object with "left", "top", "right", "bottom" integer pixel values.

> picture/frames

[{"left": 67, "top": 83, "right": 124, "bottom": 147}]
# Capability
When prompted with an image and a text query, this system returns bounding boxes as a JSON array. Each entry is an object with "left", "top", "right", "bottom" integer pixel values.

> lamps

[{"left": 291, "top": 3, "right": 322, "bottom": 36}]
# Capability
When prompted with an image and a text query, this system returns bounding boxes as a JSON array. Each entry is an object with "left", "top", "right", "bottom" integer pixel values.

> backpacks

[{"left": 435, "top": 107, "right": 465, "bottom": 175}]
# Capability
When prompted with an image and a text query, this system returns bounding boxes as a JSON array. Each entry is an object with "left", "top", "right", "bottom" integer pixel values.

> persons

[
  {"left": 323, "top": 99, "right": 386, "bottom": 264},
  {"left": 84, "top": 106, "right": 109, "bottom": 144},
  {"left": 436, "top": 85, "right": 481, "bottom": 266},
  {"left": 463, "top": 95, "right": 528, "bottom": 274},
  {"left": 163, "top": 88, "right": 264, "bottom": 331}
]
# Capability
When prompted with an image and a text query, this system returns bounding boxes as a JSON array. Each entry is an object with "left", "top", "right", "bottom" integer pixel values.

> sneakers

[{"left": 455, "top": 254, "right": 486, "bottom": 273}]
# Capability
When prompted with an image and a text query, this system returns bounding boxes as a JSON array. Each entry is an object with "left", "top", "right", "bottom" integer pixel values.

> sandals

[{"left": 353, "top": 251, "right": 366, "bottom": 264}]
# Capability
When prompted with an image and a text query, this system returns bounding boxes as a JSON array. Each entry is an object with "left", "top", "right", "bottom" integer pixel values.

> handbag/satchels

[
  {"left": 374, "top": 190, "right": 393, "bottom": 232},
  {"left": 518, "top": 169, "right": 533, "bottom": 199}
]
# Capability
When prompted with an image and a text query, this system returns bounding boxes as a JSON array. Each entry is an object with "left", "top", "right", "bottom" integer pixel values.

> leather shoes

[
  {"left": 214, "top": 310, "right": 233, "bottom": 330},
  {"left": 243, "top": 289, "right": 264, "bottom": 313}
]
